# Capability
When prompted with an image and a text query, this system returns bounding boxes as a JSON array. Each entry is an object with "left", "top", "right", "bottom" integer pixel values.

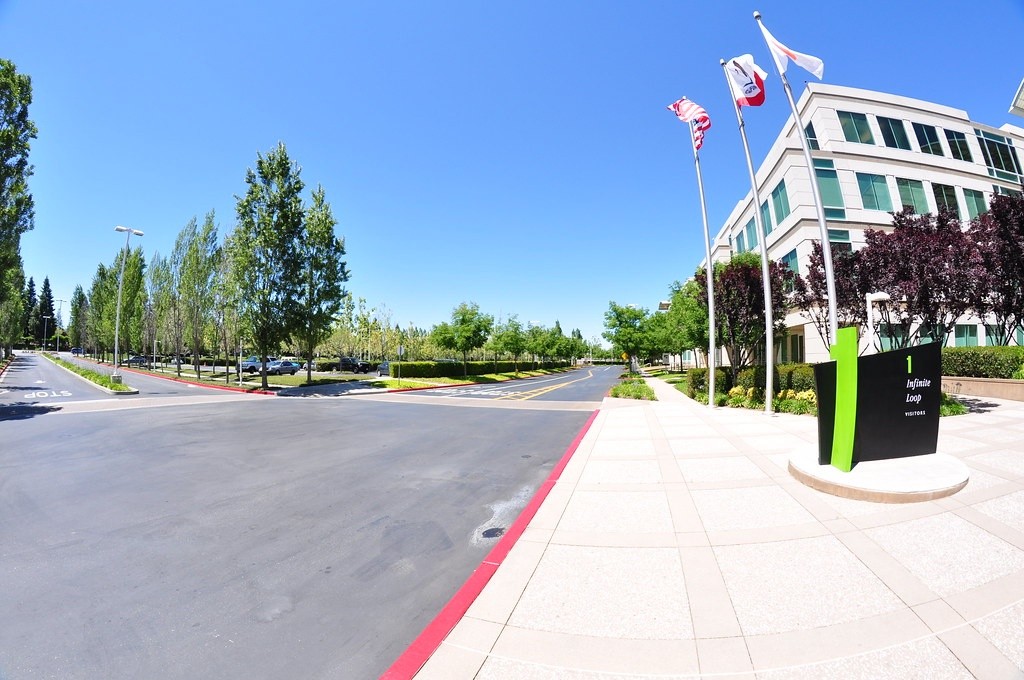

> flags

[
  {"left": 758, "top": 20, "right": 824, "bottom": 81},
  {"left": 666, "top": 99, "right": 712, "bottom": 151},
  {"left": 726, "top": 53, "right": 768, "bottom": 109}
]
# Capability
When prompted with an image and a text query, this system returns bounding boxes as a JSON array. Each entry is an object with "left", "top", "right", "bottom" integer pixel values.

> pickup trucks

[{"left": 329, "top": 357, "right": 369, "bottom": 374}]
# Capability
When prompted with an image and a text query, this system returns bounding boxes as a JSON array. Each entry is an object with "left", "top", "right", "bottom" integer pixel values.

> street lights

[
  {"left": 55, "top": 300, "right": 67, "bottom": 351},
  {"left": 42, "top": 315, "right": 51, "bottom": 351},
  {"left": 113, "top": 226, "right": 144, "bottom": 375}
]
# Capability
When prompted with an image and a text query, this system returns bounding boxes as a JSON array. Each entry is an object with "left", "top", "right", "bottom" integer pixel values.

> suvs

[
  {"left": 236, "top": 356, "right": 278, "bottom": 375},
  {"left": 71, "top": 347, "right": 86, "bottom": 355}
]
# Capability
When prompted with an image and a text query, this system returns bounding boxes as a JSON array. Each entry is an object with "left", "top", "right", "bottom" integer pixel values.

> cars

[
  {"left": 376, "top": 361, "right": 390, "bottom": 377},
  {"left": 122, "top": 356, "right": 146, "bottom": 364},
  {"left": 303, "top": 361, "right": 319, "bottom": 371},
  {"left": 259, "top": 361, "right": 299, "bottom": 378}
]
